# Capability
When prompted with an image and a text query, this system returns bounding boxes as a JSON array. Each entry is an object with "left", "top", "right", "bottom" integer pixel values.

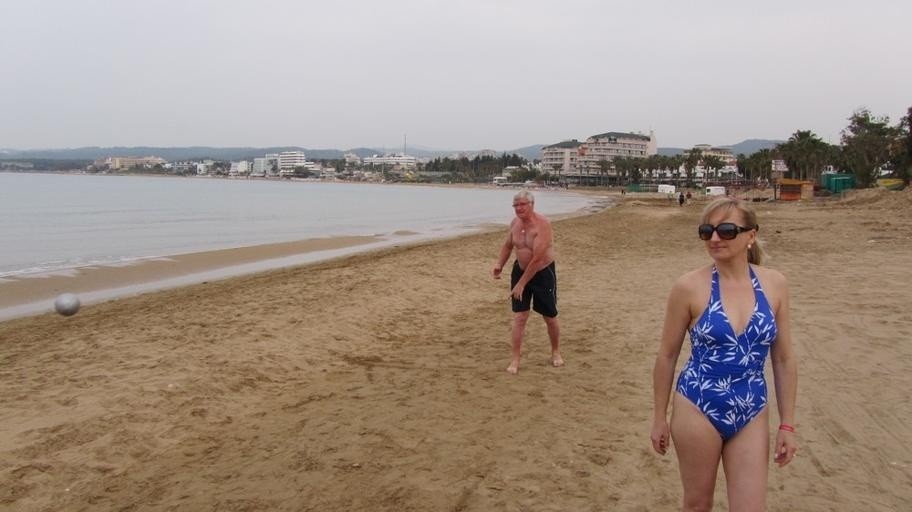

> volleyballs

[{"left": 55, "top": 293, "right": 79, "bottom": 316}]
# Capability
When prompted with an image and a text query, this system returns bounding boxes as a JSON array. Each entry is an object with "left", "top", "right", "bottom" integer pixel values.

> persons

[
  {"left": 493, "top": 189, "right": 564, "bottom": 375},
  {"left": 649, "top": 195, "right": 799, "bottom": 511},
  {"left": 668, "top": 191, "right": 673, "bottom": 206},
  {"left": 679, "top": 192, "right": 685, "bottom": 207},
  {"left": 686, "top": 191, "right": 692, "bottom": 206}
]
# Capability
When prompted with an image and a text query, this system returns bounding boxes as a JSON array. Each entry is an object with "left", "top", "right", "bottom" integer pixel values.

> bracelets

[{"left": 778, "top": 424, "right": 797, "bottom": 432}]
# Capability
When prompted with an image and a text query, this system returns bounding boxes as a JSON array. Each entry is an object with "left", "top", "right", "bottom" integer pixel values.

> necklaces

[{"left": 521, "top": 228, "right": 527, "bottom": 234}]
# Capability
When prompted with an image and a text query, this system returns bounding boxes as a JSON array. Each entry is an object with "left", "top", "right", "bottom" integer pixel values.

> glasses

[{"left": 697, "top": 223, "right": 753, "bottom": 241}]
